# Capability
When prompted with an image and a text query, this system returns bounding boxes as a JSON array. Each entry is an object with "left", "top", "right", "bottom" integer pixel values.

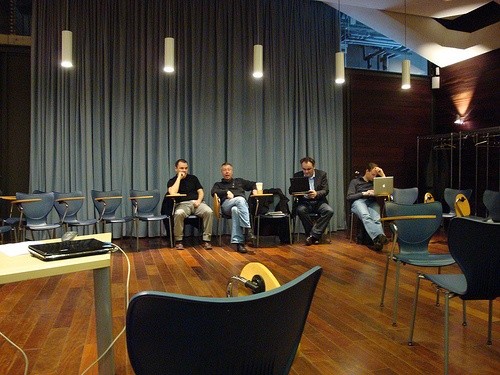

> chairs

[
  {"left": 0, "top": 177, "right": 332, "bottom": 252},
  {"left": 126, "top": 261, "right": 323, "bottom": 375},
  {"left": 347, "top": 179, "right": 500, "bottom": 375}
]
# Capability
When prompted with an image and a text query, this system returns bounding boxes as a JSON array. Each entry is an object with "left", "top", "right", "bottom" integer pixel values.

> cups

[{"left": 255, "top": 182, "right": 263, "bottom": 190}]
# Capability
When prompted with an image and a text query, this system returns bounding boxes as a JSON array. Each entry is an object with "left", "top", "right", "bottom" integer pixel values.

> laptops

[
  {"left": 28, "top": 238, "right": 113, "bottom": 261},
  {"left": 289, "top": 177, "right": 312, "bottom": 194},
  {"left": 369, "top": 176, "right": 394, "bottom": 195}
]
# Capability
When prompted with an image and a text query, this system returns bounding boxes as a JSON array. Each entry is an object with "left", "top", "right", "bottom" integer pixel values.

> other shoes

[
  {"left": 304, "top": 235, "right": 320, "bottom": 246},
  {"left": 246, "top": 230, "right": 256, "bottom": 241},
  {"left": 374, "top": 234, "right": 388, "bottom": 252},
  {"left": 237, "top": 244, "right": 248, "bottom": 253},
  {"left": 202, "top": 242, "right": 213, "bottom": 250},
  {"left": 176, "top": 243, "right": 184, "bottom": 250}
]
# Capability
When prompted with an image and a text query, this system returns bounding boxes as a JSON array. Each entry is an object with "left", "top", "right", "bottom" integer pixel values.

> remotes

[{"left": 61, "top": 230, "right": 78, "bottom": 242}]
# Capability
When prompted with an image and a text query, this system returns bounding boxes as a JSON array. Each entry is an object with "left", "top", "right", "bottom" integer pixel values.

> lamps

[
  {"left": 164, "top": 0, "right": 174, "bottom": 72},
  {"left": 402, "top": 0, "right": 410, "bottom": 89},
  {"left": 335, "top": 0, "right": 345, "bottom": 83},
  {"left": 253, "top": 0, "right": 263, "bottom": 77},
  {"left": 453, "top": 114, "right": 464, "bottom": 124},
  {"left": 61, "top": 0, "right": 72, "bottom": 67}
]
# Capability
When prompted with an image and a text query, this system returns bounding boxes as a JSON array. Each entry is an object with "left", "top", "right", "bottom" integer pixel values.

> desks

[{"left": 0, "top": 231, "right": 114, "bottom": 375}]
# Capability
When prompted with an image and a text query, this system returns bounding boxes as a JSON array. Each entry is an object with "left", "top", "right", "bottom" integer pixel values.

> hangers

[
  {"left": 475, "top": 134, "right": 500, "bottom": 147},
  {"left": 434, "top": 138, "right": 455, "bottom": 149}
]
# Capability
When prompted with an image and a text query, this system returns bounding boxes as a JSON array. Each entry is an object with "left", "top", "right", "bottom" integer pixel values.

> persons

[
  {"left": 346, "top": 162, "right": 389, "bottom": 252},
  {"left": 211, "top": 162, "right": 256, "bottom": 253},
  {"left": 289, "top": 157, "right": 334, "bottom": 245},
  {"left": 166, "top": 159, "right": 213, "bottom": 250}
]
